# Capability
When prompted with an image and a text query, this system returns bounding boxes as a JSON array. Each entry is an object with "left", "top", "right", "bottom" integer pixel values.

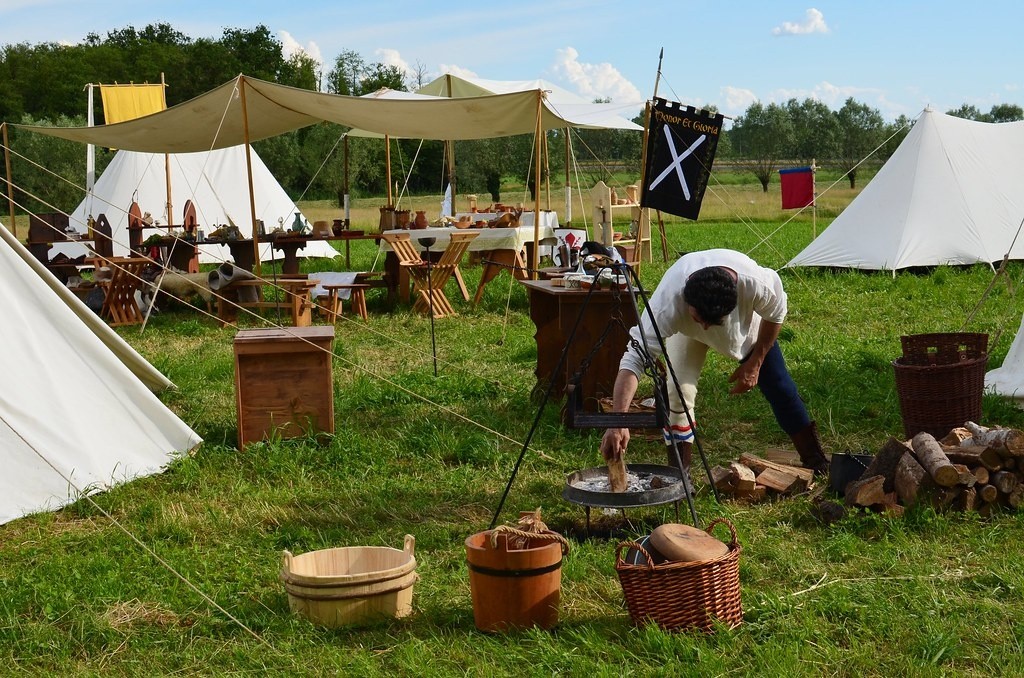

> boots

[
  {"left": 789, "top": 421, "right": 830, "bottom": 476},
  {"left": 666, "top": 442, "right": 697, "bottom": 498}
]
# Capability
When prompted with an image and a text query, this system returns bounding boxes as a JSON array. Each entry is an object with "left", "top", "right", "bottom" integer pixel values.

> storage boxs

[{"left": 29, "top": 213, "right": 69, "bottom": 242}]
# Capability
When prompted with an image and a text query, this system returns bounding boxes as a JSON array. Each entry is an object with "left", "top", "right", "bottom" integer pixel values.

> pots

[{"left": 565, "top": 464, "right": 688, "bottom": 506}]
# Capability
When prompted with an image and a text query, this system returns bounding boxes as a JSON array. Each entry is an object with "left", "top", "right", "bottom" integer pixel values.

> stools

[{"left": 322, "top": 284, "right": 371, "bottom": 325}]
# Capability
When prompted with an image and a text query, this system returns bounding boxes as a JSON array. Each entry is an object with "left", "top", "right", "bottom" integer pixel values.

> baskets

[
  {"left": 614, "top": 518, "right": 743, "bottom": 639},
  {"left": 892, "top": 332, "right": 990, "bottom": 441}
]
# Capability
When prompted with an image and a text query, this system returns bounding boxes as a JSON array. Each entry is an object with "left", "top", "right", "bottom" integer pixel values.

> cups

[
  {"left": 476, "top": 221, "right": 482, "bottom": 228},
  {"left": 198, "top": 229, "right": 204, "bottom": 242},
  {"left": 516, "top": 203, "right": 523, "bottom": 210},
  {"left": 470, "top": 196, "right": 476, "bottom": 213}
]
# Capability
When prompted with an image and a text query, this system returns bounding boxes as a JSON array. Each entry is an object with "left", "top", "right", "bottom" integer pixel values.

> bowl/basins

[
  {"left": 614, "top": 233, "right": 622, "bottom": 240},
  {"left": 478, "top": 210, "right": 487, "bottom": 213},
  {"left": 455, "top": 221, "right": 470, "bottom": 228},
  {"left": 280, "top": 534, "right": 417, "bottom": 627},
  {"left": 618, "top": 199, "right": 627, "bottom": 204}
]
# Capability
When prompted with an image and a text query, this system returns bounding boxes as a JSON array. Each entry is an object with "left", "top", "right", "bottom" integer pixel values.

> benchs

[
  {"left": 260, "top": 272, "right": 382, "bottom": 323},
  {"left": 217, "top": 279, "right": 316, "bottom": 330}
]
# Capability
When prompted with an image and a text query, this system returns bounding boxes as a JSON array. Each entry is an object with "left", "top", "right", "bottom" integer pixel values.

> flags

[{"left": 640, "top": 97, "right": 724, "bottom": 221}]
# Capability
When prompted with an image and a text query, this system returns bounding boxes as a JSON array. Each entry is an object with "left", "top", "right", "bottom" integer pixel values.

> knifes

[{"left": 602, "top": 210, "right": 606, "bottom": 245}]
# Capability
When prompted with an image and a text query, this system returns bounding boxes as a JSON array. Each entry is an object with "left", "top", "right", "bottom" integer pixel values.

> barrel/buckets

[{"left": 465, "top": 512, "right": 563, "bottom": 634}]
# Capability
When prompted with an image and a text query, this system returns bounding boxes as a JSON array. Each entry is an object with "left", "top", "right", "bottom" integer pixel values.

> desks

[
  {"left": 180, "top": 239, "right": 254, "bottom": 273},
  {"left": 259, "top": 234, "right": 381, "bottom": 274},
  {"left": 518, "top": 278, "right": 651, "bottom": 424},
  {"left": 233, "top": 326, "right": 336, "bottom": 452},
  {"left": 379, "top": 225, "right": 556, "bottom": 312},
  {"left": 455, "top": 211, "right": 559, "bottom": 263}
]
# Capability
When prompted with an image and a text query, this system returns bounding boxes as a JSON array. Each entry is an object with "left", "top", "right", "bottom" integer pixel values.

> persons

[{"left": 600, "top": 248, "right": 832, "bottom": 477}]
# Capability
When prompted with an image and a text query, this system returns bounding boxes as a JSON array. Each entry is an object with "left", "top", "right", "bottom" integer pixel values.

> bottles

[
  {"left": 576, "top": 257, "right": 586, "bottom": 275},
  {"left": 292, "top": 213, "right": 305, "bottom": 231},
  {"left": 612, "top": 185, "right": 617, "bottom": 205}
]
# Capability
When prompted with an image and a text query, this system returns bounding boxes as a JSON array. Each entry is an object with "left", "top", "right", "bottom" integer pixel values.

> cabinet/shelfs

[
  {"left": 26, "top": 213, "right": 113, "bottom": 310},
  {"left": 125, "top": 199, "right": 200, "bottom": 273},
  {"left": 590, "top": 179, "right": 653, "bottom": 264}
]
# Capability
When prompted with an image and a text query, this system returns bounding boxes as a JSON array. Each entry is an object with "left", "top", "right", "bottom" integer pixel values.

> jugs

[{"left": 332, "top": 220, "right": 345, "bottom": 235}]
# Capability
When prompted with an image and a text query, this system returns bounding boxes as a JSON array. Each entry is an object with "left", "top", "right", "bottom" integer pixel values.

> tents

[
  {"left": 0, "top": 72, "right": 650, "bottom": 528},
  {"left": 775, "top": 104, "right": 1024, "bottom": 278}
]
